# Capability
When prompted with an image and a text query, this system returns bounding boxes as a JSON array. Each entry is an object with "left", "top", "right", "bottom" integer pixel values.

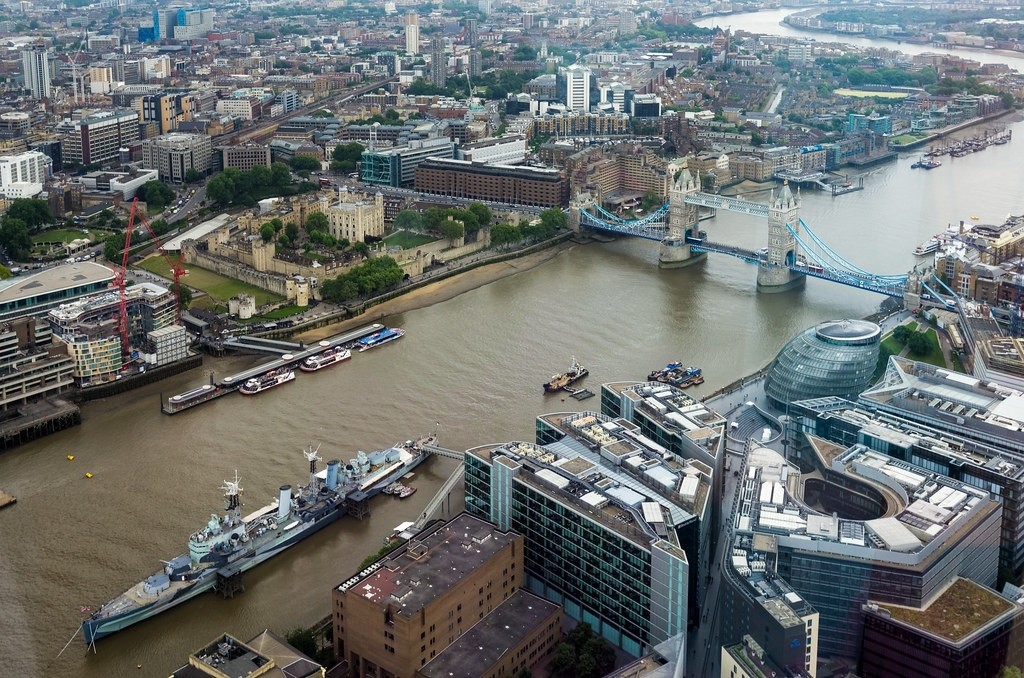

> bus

[
  {"left": 687, "top": 236, "right": 703, "bottom": 244},
  {"left": 808, "top": 265, "right": 824, "bottom": 274}
]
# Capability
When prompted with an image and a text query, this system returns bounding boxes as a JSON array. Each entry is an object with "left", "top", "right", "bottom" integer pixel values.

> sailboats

[{"left": 842, "top": 173, "right": 852, "bottom": 187}]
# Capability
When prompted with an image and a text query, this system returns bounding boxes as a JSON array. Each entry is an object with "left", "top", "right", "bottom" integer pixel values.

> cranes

[{"left": 109, "top": 199, "right": 190, "bottom": 372}]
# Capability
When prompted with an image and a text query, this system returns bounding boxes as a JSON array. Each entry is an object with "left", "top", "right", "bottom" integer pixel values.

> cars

[
  {"left": 64, "top": 249, "right": 103, "bottom": 265},
  {"left": 163, "top": 189, "right": 196, "bottom": 217}
]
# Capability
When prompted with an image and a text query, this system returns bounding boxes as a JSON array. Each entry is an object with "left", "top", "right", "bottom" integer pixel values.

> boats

[
  {"left": 648, "top": 361, "right": 705, "bottom": 387},
  {"left": 237, "top": 365, "right": 296, "bottom": 395},
  {"left": 300, "top": 344, "right": 352, "bottom": 373},
  {"left": 910, "top": 129, "right": 1010, "bottom": 170},
  {"left": 540, "top": 355, "right": 589, "bottom": 392},
  {"left": 353, "top": 327, "right": 407, "bottom": 352},
  {"left": 81, "top": 436, "right": 439, "bottom": 645},
  {"left": 912, "top": 222, "right": 971, "bottom": 256}
]
